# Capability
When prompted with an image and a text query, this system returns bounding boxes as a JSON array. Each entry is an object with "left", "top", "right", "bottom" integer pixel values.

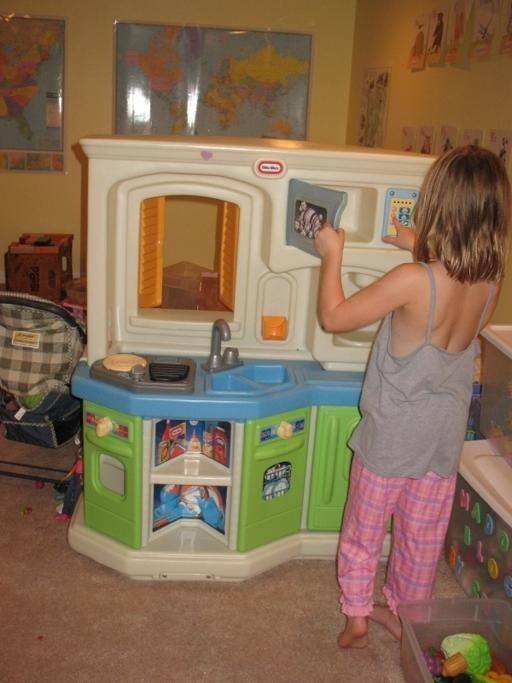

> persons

[{"left": 313, "top": 144, "right": 512, "bottom": 647}]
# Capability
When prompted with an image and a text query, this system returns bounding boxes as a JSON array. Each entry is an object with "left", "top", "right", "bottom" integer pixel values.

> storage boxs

[
  {"left": 443, "top": 440, "right": 511, "bottom": 662},
  {"left": 399, "top": 596, "right": 509, "bottom": 682},
  {"left": 474, "top": 324, "right": 511, "bottom": 469}
]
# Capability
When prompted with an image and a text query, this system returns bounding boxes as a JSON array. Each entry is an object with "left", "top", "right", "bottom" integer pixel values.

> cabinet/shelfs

[
  {"left": 285, "top": 169, "right": 425, "bottom": 260},
  {"left": 67, "top": 399, "right": 393, "bottom": 583}
]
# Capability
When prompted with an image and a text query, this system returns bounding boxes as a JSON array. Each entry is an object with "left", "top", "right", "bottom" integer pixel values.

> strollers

[{"left": 0, "top": 289, "right": 88, "bottom": 516}]
